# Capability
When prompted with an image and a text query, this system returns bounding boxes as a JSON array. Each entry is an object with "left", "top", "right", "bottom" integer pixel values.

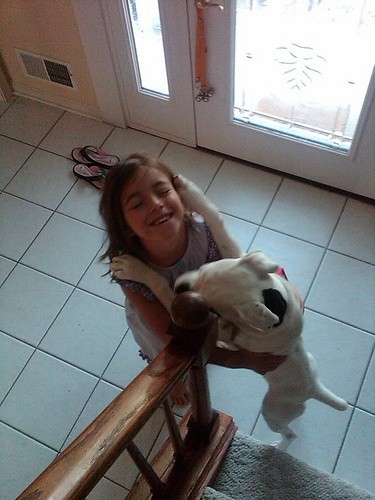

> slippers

[
  {"left": 73, "top": 163, "right": 110, "bottom": 189},
  {"left": 72, "top": 145, "right": 120, "bottom": 168}
]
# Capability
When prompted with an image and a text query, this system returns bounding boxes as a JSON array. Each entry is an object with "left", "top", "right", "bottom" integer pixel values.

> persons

[{"left": 99, "top": 154, "right": 304, "bottom": 407}]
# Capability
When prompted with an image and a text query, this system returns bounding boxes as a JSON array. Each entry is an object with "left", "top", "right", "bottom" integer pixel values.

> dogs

[{"left": 111, "top": 178, "right": 348, "bottom": 451}]
